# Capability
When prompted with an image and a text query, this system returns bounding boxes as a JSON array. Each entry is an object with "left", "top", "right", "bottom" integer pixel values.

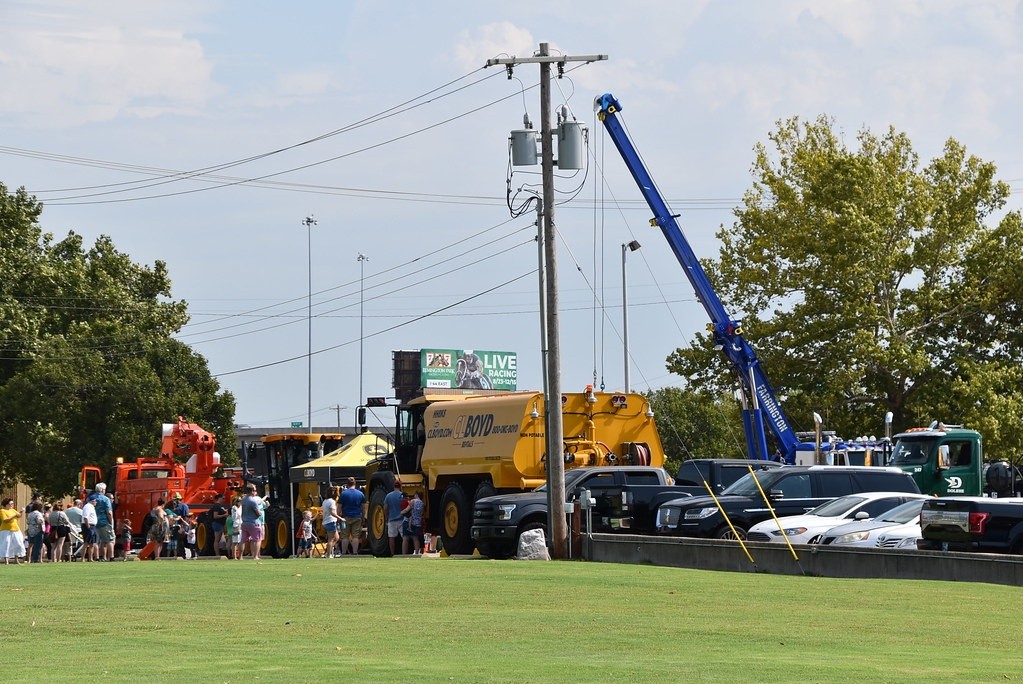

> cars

[
  {"left": 746, "top": 491, "right": 931, "bottom": 543},
  {"left": 234, "top": 423, "right": 251, "bottom": 429},
  {"left": 818, "top": 496, "right": 936, "bottom": 549}
]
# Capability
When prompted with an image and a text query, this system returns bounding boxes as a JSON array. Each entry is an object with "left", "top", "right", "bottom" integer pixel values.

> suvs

[
  {"left": 656, "top": 463, "right": 922, "bottom": 540},
  {"left": 471, "top": 465, "right": 677, "bottom": 561}
]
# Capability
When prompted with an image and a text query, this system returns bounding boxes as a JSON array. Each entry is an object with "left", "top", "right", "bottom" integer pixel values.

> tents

[{"left": 289, "top": 430, "right": 396, "bottom": 556}]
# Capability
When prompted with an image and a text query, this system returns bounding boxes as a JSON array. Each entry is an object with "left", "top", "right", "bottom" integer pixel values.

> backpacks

[{"left": 205, "top": 505, "right": 221, "bottom": 523}]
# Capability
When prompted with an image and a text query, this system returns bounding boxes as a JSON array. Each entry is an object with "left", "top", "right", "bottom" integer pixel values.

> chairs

[
  {"left": 956, "top": 443, "right": 971, "bottom": 464},
  {"left": 311, "top": 526, "right": 327, "bottom": 556}
]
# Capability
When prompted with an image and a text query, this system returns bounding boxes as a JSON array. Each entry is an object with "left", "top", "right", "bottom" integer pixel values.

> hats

[
  {"left": 86, "top": 495, "right": 97, "bottom": 502},
  {"left": 173, "top": 494, "right": 183, "bottom": 500}
]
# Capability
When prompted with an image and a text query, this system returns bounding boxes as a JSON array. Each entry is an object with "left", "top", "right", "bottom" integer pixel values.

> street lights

[
  {"left": 302, "top": 216, "right": 318, "bottom": 434},
  {"left": 357, "top": 252, "right": 370, "bottom": 402},
  {"left": 621, "top": 239, "right": 642, "bottom": 392}
]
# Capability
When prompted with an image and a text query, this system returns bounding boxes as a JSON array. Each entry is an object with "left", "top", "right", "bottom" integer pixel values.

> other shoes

[
  {"left": 406, "top": 527, "right": 414, "bottom": 533},
  {"left": 95, "top": 556, "right": 115, "bottom": 562}
]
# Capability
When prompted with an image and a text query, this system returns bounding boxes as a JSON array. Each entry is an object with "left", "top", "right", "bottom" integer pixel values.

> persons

[
  {"left": 322, "top": 487, "right": 347, "bottom": 559},
  {"left": 906, "top": 444, "right": 924, "bottom": 459},
  {"left": 338, "top": 477, "right": 366, "bottom": 555},
  {"left": 384, "top": 480, "right": 426, "bottom": 558},
  {"left": 211, "top": 494, "right": 229, "bottom": 556},
  {"left": 295, "top": 511, "right": 319, "bottom": 558},
  {"left": 26, "top": 482, "right": 116, "bottom": 563},
  {"left": 225, "top": 483, "right": 270, "bottom": 561},
  {"left": 0, "top": 498, "right": 27, "bottom": 565},
  {"left": 121, "top": 519, "right": 134, "bottom": 561},
  {"left": 146, "top": 492, "right": 197, "bottom": 560}
]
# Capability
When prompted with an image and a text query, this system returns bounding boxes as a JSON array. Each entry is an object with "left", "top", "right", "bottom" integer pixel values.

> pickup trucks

[
  {"left": 919, "top": 493, "right": 1023, "bottom": 553},
  {"left": 577, "top": 457, "right": 784, "bottom": 534}
]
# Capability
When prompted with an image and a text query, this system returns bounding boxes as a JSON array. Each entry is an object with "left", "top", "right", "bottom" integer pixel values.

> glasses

[{"left": 110, "top": 497, "right": 114, "bottom": 499}]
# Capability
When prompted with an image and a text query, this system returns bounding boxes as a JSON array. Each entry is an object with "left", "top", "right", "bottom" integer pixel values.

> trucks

[{"left": 886, "top": 423, "right": 1023, "bottom": 497}]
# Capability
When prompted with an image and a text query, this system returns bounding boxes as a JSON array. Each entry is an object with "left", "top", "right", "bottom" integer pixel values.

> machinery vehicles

[
  {"left": 195, "top": 432, "right": 346, "bottom": 558},
  {"left": 593, "top": 92, "right": 890, "bottom": 465},
  {"left": 355, "top": 390, "right": 667, "bottom": 559},
  {"left": 80, "top": 416, "right": 256, "bottom": 541}
]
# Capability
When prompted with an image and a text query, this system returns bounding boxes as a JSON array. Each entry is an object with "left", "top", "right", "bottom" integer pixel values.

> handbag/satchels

[{"left": 57, "top": 525, "right": 66, "bottom": 537}]
